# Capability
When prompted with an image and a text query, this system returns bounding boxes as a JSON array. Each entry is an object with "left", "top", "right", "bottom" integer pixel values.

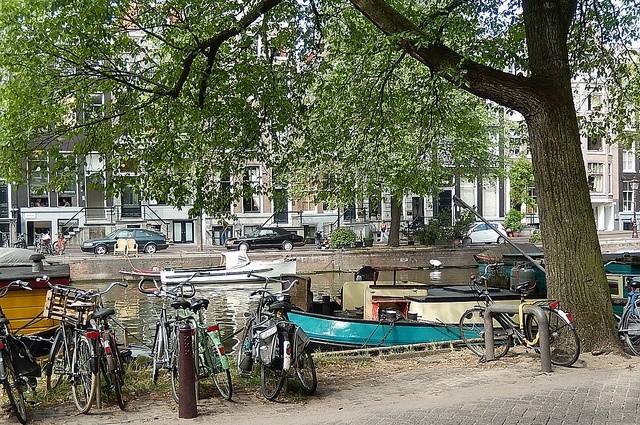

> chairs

[
  {"left": 126, "top": 239, "right": 139, "bottom": 257},
  {"left": 113, "top": 238, "right": 126, "bottom": 255}
]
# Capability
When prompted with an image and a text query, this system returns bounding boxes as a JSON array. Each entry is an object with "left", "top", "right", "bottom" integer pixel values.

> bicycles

[
  {"left": 459, "top": 262, "right": 579, "bottom": 365},
  {"left": 170, "top": 272, "right": 233, "bottom": 400},
  {"left": 34, "top": 230, "right": 53, "bottom": 254},
  {"left": 251, "top": 279, "right": 317, "bottom": 393},
  {"left": 36, "top": 275, "right": 100, "bottom": 413},
  {"left": 53, "top": 230, "right": 65, "bottom": 253},
  {"left": 239, "top": 270, "right": 294, "bottom": 399},
  {"left": 0, "top": 279, "right": 41, "bottom": 424},
  {"left": 138, "top": 277, "right": 199, "bottom": 404},
  {"left": 74, "top": 281, "right": 129, "bottom": 409},
  {"left": 604, "top": 260, "right": 640, "bottom": 355}
]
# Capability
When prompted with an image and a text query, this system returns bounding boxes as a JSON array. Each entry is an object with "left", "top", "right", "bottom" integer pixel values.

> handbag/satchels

[
  {"left": 250, "top": 319, "right": 280, "bottom": 367},
  {"left": 291, "top": 325, "right": 311, "bottom": 368},
  {"left": 6, "top": 333, "right": 41, "bottom": 377}
]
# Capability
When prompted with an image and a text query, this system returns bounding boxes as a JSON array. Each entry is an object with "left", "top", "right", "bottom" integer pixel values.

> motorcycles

[
  {"left": 14, "top": 231, "right": 27, "bottom": 247},
  {"left": 315, "top": 230, "right": 330, "bottom": 248}
]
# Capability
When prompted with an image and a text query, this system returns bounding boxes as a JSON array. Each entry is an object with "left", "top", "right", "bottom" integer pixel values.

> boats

[
  {"left": 286, "top": 194, "right": 640, "bottom": 346},
  {"left": 0, "top": 246, "right": 70, "bottom": 374},
  {"left": 120, "top": 254, "right": 297, "bottom": 282}
]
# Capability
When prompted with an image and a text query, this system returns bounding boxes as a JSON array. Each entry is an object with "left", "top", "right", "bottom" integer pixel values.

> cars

[
  {"left": 225, "top": 227, "right": 303, "bottom": 250},
  {"left": 463, "top": 221, "right": 508, "bottom": 243},
  {"left": 81, "top": 227, "right": 168, "bottom": 254}
]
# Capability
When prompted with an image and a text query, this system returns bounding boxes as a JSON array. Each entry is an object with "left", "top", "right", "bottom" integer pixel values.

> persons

[{"left": 380, "top": 220, "right": 388, "bottom": 243}]
